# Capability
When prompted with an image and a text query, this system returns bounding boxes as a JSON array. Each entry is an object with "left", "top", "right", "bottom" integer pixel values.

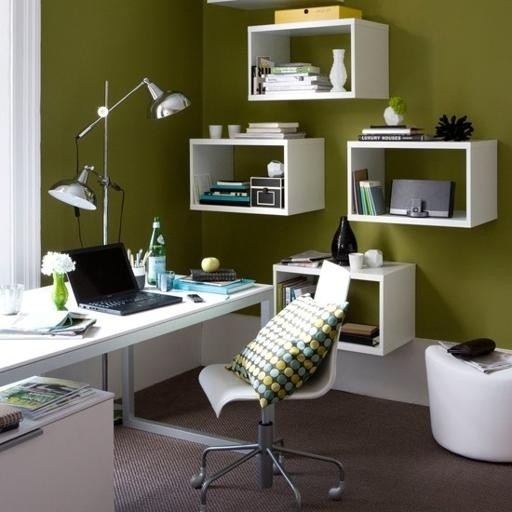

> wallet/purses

[{"left": 448, "top": 338, "right": 498, "bottom": 359}]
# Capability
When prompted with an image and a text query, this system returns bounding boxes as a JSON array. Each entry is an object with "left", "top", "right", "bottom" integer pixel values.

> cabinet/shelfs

[{"left": 0, "top": 374, "right": 123, "bottom": 512}]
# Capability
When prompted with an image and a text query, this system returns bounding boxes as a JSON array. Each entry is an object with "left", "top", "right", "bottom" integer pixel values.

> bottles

[
  {"left": 147, "top": 216, "right": 168, "bottom": 285},
  {"left": 330, "top": 215, "right": 358, "bottom": 265}
]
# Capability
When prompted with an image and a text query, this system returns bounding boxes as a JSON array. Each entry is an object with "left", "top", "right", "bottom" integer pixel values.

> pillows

[{"left": 223, "top": 290, "right": 350, "bottom": 413}]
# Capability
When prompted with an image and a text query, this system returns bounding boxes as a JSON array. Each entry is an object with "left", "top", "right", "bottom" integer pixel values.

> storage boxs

[
  {"left": 248, "top": 176, "right": 286, "bottom": 209},
  {"left": 273, "top": 5, "right": 363, "bottom": 23}
]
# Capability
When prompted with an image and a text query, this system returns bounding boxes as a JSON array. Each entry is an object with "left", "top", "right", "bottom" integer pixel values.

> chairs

[{"left": 190, "top": 255, "right": 357, "bottom": 512}]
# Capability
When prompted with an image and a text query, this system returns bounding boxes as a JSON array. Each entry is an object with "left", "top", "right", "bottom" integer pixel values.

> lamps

[{"left": 44, "top": 70, "right": 196, "bottom": 245}]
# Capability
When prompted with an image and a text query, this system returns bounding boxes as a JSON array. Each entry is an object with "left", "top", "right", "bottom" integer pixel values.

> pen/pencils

[{"left": 127, "top": 248, "right": 152, "bottom": 268}]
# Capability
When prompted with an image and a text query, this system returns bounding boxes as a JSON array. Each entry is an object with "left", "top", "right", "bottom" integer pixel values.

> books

[
  {"left": 338, "top": 331, "right": 382, "bottom": 346},
  {"left": 235, "top": 120, "right": 305, "bottom": 141},
  {"left": 172, "top": 269, "right": 257, "bottom": 294},
  {"left": 357, "top": 124, "right": 434, "bottom": 142},
  {"left": 251, "top": 61, "right": 334, "bottom": 96},
  {"left": 351, "top": 167, "right": 386, "bottom": 215},
  {"left": 199, "top": 179, "right": 253, "bottom": 207},
  {"left": 277, "top": 275, "right": 316, "bottom": 314},
  {"left": 0, "top": 306, "right": 99, "bottom": 341},
  {"left": 341, "top": 322, "right": 377, "bottom": 336},
  {"left": 1, "top": 376, "right": 98, "bottom": 435}
]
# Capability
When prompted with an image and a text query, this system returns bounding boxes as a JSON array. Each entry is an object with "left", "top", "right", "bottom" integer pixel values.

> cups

[
  {"left": 1, "top": 284, "right": 23, "bottom": 316},
  {"left": 208, "top": 125, "right": 224, "bottom": 140},
  {"left": 347, "top": 252, "right": 363, "bottom": 270},
  {"left": 132, "top": 265, "right": 146, "bottom": 290},
  {"left": 155, "top": 271, "right": 176, "bottom": 291},
  {"left": 227, "top": 124, "right": 241, "bottom": 140}
]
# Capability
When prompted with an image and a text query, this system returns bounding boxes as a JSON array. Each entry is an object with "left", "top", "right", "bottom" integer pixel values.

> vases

[{"left": 51, "top": 271, "right": 69, "bottom": 311}]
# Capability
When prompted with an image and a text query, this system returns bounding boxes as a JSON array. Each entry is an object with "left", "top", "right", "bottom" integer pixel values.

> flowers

[{"left": 39, "top": 248, "right": 80, "bottom": 279}]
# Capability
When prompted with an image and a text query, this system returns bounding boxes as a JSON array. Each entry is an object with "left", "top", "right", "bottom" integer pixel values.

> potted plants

[{"left": 382, "top": 96, "right": 409, "bottom": 128}]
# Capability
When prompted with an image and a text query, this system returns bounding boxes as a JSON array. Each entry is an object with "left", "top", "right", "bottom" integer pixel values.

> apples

[{"left": 201, "top": 257, "right": 220, "bottom": 272}]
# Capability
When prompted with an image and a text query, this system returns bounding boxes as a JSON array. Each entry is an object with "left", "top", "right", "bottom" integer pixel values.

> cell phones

[{"left": 188, "top": 293, "right": 203, "bottom": 302}]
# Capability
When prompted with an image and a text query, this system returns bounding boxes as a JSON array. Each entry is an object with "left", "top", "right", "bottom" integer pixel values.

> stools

[{"left": 420, "top": 340, "right": 511, "bottom": 462}]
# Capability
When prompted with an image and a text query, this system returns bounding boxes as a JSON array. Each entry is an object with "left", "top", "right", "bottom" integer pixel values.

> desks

[{"left": 0, "top": 269, "right": 282, "bottom": 511}]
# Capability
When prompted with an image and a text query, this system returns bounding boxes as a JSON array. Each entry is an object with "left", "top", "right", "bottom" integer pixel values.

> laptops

[{"left": 61, "top": 242, "right": 182, "bottom": 316}]
